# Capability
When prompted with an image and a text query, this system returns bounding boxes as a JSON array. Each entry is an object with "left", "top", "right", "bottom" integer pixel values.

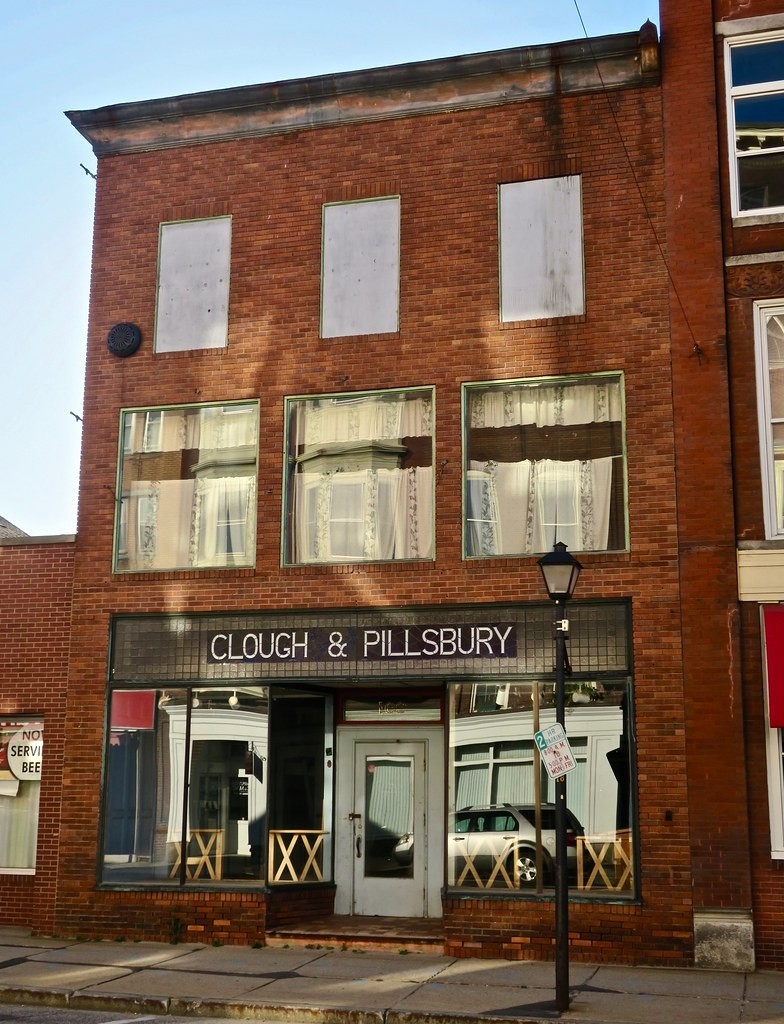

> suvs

[{"left": 392, "top": 801, "right": 586, "bottom": 888}]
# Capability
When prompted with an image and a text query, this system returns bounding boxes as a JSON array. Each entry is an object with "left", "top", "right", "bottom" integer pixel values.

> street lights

[{"left": 537, "top": 541, "right": 585, "bottom": 1014}]
